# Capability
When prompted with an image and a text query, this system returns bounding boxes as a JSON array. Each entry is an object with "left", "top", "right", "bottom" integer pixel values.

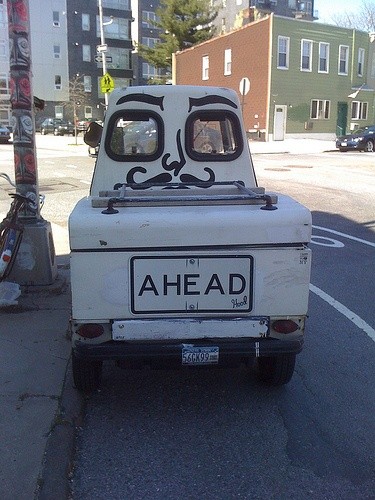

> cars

[
  {"left": 336, "top": 125, "right": 375, "bottom": 152},
  {"left": 88, "top": 117, "right": 223, "bottom": 158},
  {"left": 0, "top": 123, "right": 11, "bottom": 142}
]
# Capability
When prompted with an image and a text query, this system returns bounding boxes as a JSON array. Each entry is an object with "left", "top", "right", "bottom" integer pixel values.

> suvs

[
  {"left": 76, "top": 118, "right": 99, "bottom": 133},
  {"left": 41, "top": 118, "right": 79, "bottom": 136}
]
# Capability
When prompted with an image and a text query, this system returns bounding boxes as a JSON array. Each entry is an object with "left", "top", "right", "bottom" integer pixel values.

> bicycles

[{"left": 0, "top": 173, "right": 46, "bottom": 282}]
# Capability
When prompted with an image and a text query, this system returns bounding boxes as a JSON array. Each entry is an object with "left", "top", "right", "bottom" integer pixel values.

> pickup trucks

[{"left": 68, "top": 84, "right": 312, "bottom": 390}]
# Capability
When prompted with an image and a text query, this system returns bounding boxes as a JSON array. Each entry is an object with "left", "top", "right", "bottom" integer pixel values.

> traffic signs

[{"left": 94, "top": 44, "right": 112, "bottom": 63}]
